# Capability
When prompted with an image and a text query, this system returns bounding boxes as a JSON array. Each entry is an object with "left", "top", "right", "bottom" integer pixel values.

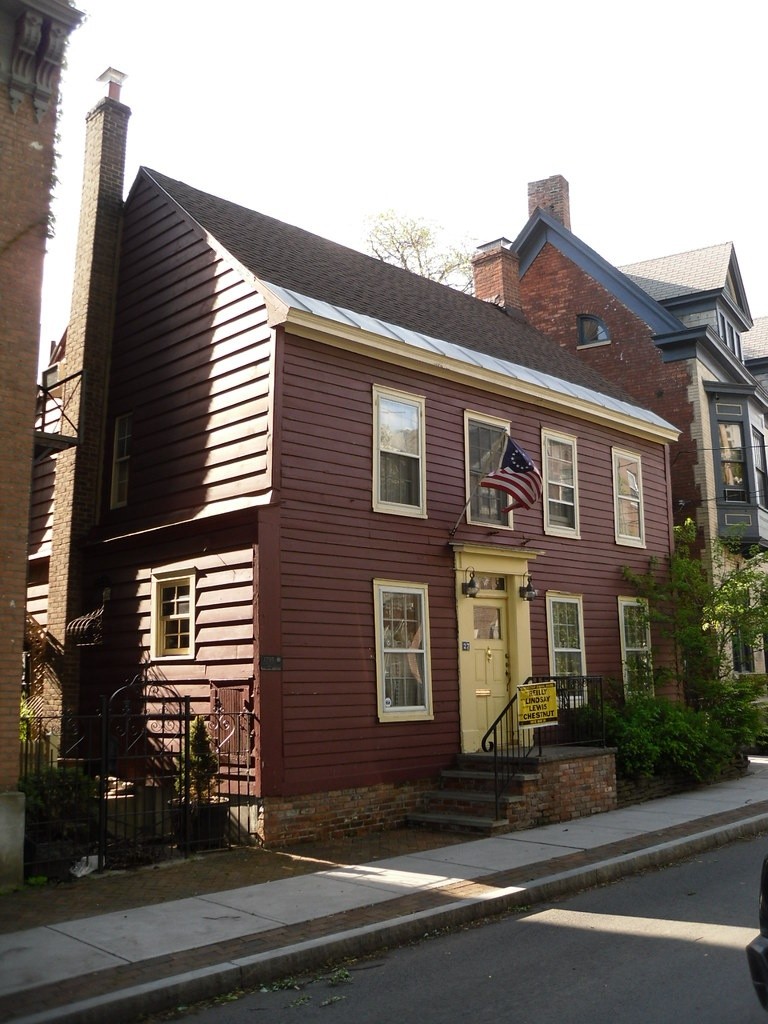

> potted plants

[{"left": 164, "top": 714, "right": 231, "bottom": 849}]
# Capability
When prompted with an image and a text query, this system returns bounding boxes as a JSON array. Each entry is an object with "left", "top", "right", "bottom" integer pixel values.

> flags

[{"left": 484, "top": 434, "right": 544, "bottom": 513}]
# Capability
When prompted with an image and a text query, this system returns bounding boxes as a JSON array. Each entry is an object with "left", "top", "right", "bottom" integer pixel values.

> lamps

[
  {"left": 463, "top": 567, "right": 480, "bottom": 598},
  {"left": 520, "top": 569, "right": 537, "bottom": 602}
]
